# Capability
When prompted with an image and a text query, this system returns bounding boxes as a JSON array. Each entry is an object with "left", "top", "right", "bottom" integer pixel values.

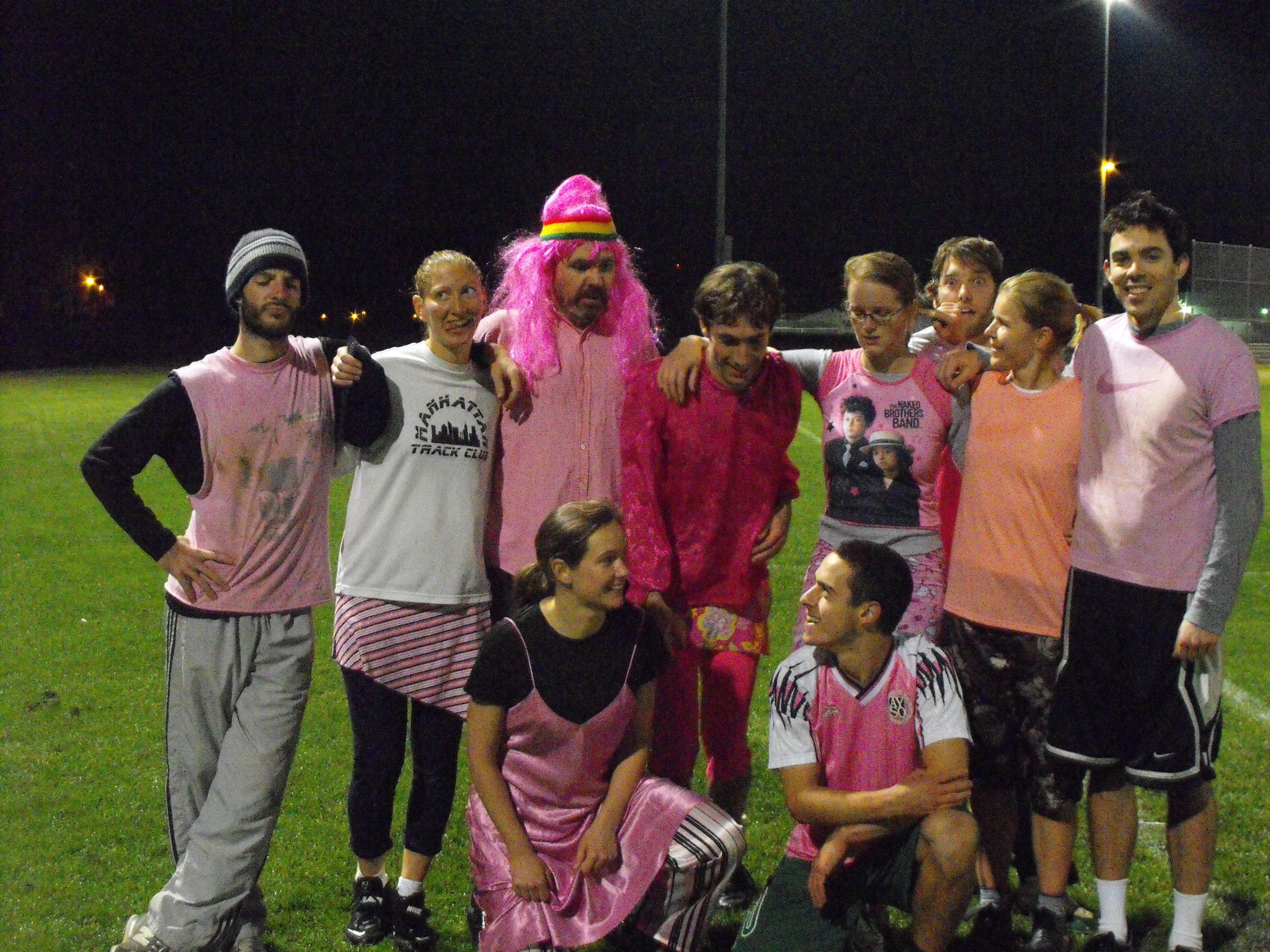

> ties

[{"left": 842, "top": 444, "right": 852, "bottom": 467}]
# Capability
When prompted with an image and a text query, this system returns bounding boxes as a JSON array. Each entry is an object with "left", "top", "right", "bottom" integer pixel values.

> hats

[
  {"left": 340, "top": 335, "right": 390, "bottom": 447},
  {"left": 223, "top": 227, "right": 311, "bottom": 313},
  {"left": 860, "top": 430, "right": 914, "bottom": 453},
  {"left": 538, "top": 173, "right": 617, "bottom": 239}
]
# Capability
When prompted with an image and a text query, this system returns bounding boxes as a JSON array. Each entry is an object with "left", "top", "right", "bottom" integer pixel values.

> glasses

[{"left": 844, "top": 301, "right": 908, "bottom": 323}]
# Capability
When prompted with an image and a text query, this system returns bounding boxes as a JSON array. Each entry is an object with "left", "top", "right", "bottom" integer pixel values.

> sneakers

[
  {"left": 110, "top": 914, "right": 172, "bottom": 952},
  {"left": 968, "top": 906, "right": 1013, "bottom": 952},
  {"left": 391, "top": 889, "right": 438, "bottom": 952},
  {"left": 345, "top": 876, "right": 390, "bottom": 945},
  {"left": 233, "top": 934, "right": 267, "bottom": 952},
  {"left": 1026, "top": 907, "right": 1075, "bottom": 952}
]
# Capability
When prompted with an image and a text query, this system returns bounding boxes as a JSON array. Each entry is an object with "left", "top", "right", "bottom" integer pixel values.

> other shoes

[
  {"left": 719, "top": 863, "right": 757, "bottom": 909},
  {"left": 848, "top": 901, "right": 886, "bottom": 952},
  {"left": 1080, "top": 932, "right": 1132, "bottom": 952},
  {"left": 468, "top": 886, "right": 487, "bottom": 941}
]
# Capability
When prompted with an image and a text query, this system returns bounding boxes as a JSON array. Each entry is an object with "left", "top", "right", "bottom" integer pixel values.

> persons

[
  {"left": 464, "top": 502, "right": 747, "bottom": 952},
  {"left": 79, "top": 228, "right": 527, "bottom": 952},
  {"left": 332, "top": 250, "right": 501, "bottom": 952},
  {"left": 471, "top": 172, "right": 800, "bottom": 909},
  {"left": 655, "top": 193, "right": 1264, "bottom": 952},
  {"left": 730, "top": 540, "right": 980, "bottom": 952}
]
